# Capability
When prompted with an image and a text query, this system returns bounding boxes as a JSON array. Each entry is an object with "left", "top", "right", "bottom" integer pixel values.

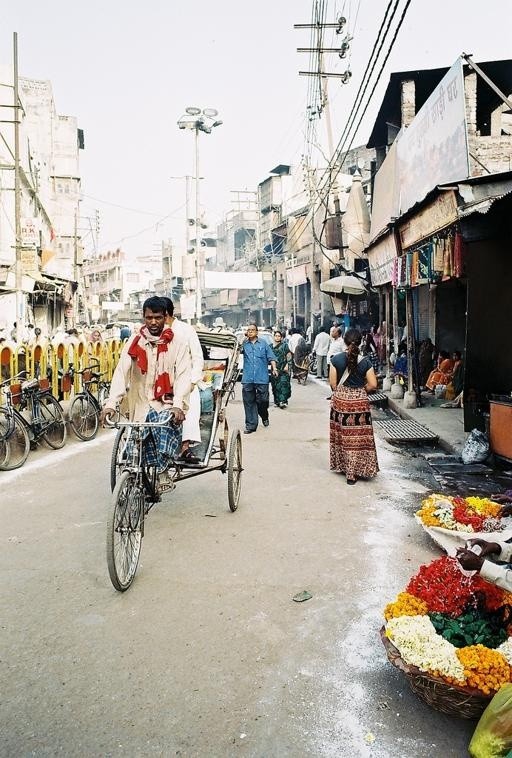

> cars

[{"left": 234, "top": 328, "right": 278, "bottom": 380}]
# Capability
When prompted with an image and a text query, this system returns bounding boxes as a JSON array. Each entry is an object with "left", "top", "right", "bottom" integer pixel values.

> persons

[
  {"left": 455, "top": 490, "right": 511, "bottom": 626},
  {"left": 397, "top": 319, "right": 462, "bottom": 401}
]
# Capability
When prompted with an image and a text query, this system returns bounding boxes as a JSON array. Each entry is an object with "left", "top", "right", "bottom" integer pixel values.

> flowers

[
  {"left": 416, "top": 492, "right": 507, "bottom": 534},
  {"left": 384, "top": 551, "right": 512, "bottom": 694}
]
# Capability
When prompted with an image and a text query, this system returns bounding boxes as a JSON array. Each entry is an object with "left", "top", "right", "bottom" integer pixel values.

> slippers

[
  {"left": 156, "top": 481, "right": 174, "bottom": 494},
  {"left": 177, "top": 449, "right": 201, "bottom": 462},
  {"left": 347, "top": 477, "right": 359, "bottom": 485}
]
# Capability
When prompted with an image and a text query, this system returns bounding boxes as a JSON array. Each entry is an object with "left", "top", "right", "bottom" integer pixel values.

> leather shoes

[
  {"left": 262, "top": 420, "right": 269, "bottom": 426},
  {"left": 244, "top": 429, "right": 251, "bottom": 433}
]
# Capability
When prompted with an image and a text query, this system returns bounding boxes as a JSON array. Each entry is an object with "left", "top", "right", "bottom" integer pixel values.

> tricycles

[{"left": 100, "top": 329, "right": 245, "bottom": 596}]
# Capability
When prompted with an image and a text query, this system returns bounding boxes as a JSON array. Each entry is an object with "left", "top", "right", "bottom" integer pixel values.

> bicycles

[
  {"left": 0, "top": 369, "right": 69, "bottom": 474},
  {"left": 292, "top": 346, "right": 310, "bottom": 386},
  {"left": 65, "top": 360, "right": 121, "bottom": 440}
]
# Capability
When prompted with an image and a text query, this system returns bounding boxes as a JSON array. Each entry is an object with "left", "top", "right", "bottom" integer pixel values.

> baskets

[{"left": 380, "top": 621, "right": 496, "bottom": 719}]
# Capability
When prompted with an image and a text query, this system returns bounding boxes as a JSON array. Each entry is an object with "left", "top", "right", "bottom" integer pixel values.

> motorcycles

[{"left": 309, "top": 350, "right": 319, "bottom": 375}]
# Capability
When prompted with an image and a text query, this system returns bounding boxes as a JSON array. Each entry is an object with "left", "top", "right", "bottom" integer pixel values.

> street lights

[{"left": 173, "top": 103, "right": 225, "bottom": 324}]
